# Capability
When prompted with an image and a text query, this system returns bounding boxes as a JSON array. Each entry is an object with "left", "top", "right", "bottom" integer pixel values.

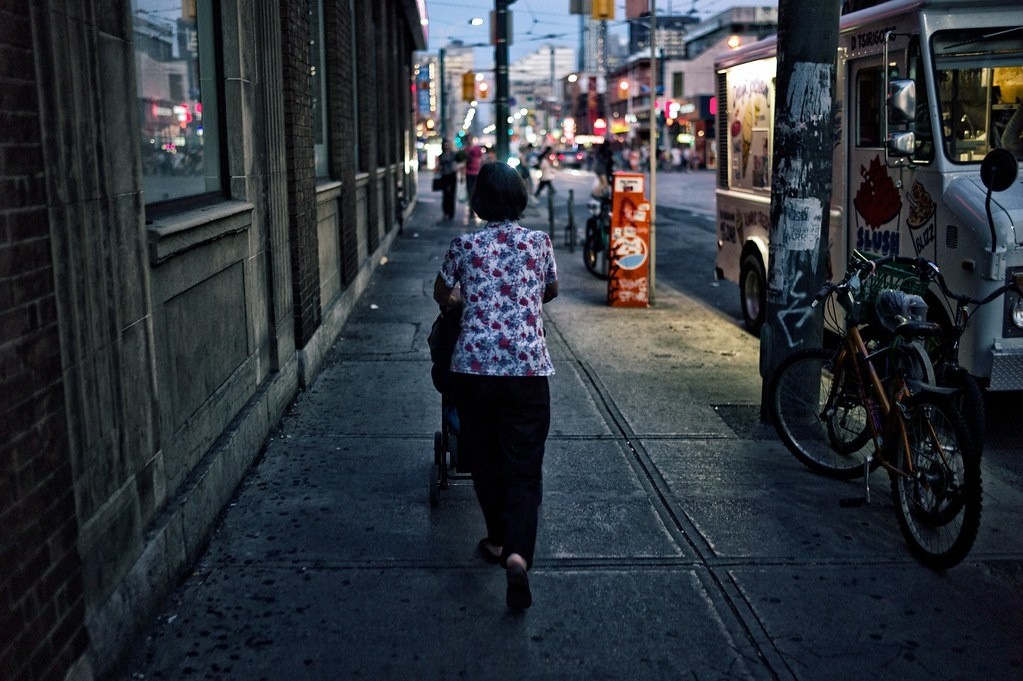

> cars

[{"left": 554, "top": 149, "right": 583, "bottom": 169}]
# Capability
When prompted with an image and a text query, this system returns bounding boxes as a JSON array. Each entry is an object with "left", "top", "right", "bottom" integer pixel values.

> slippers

[
  {"left": 505, "top": 565, "right": 533, "bottom": 610},
  {"left": 479, "top": 537, "right": 503, "bottom": 562}
]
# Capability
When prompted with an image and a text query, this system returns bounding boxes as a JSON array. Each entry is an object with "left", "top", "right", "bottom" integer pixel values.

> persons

[
  {"left": 598, "top": 140, "right": 614, "bottom": 184},
  {"left": 433, "top": 162, "right": 559, "bottom": 608},
  {"left": 519, "top": 143, "right": 533, "bottom": 192},
  {"left": 438, "top": 140, "right": 458, "bottom": 221},
  {"left": 535, "top": 147, "right": 556, "bottom": 197},
  {"left": 462, "top": 134, "right": 483, "bottom": 217}
]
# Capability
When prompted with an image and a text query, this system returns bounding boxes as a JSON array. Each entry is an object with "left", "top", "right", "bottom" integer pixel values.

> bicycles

[
  {"left": 763, "top": 249, "right": 985, "bottom": 575},
  {"left": 582, "top": 186, "right": 614, "bottom": 281},
  {"left": 819, "top": 254, "right": 1021, "bottom": 529}
]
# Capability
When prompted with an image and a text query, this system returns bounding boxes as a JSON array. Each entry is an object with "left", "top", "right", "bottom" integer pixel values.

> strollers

[{"left": 427, "top": 306, "right": 483, "bottom": 506}]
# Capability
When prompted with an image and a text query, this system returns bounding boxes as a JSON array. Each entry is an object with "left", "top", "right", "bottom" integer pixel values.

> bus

[{"left": 711, "top": 1, "right": 1023, "bottom": 391}]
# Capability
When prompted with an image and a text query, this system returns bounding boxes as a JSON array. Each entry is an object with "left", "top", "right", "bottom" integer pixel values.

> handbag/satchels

[{"left": 433, "top": 179, "right": 442, "bottom": 191}]
